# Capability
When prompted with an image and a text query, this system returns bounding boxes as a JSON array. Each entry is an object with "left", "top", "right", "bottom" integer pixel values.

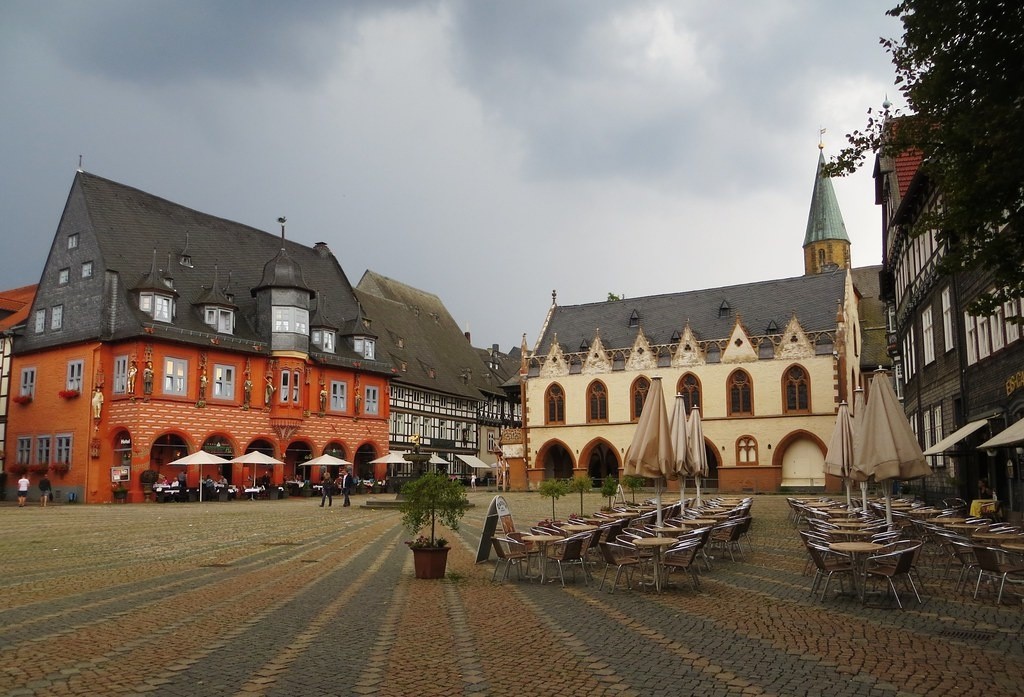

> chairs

[
  {"left": 491, "top": 495, "right": 754, "bottom": 594},
  {"left": 784, "top": 496, "right": 1024, "bottom": 609}
]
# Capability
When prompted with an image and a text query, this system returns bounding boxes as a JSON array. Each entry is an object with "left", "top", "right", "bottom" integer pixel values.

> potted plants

[{"left": 139, "top": 469, "right": 159, "bottom": 495}]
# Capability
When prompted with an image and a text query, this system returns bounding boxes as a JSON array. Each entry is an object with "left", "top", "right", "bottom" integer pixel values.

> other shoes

[
  {"left": 319, "top": 504, "right": 324, "bottom": 507},
  {"left": 343, "top": 504, "right": 347, "bottom": 507},
  {"left": 347, "top": 504, "right": 350, "bottom": 506}
]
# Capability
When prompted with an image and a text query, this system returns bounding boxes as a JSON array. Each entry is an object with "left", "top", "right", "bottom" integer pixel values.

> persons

[
  {"left": 244, "top": 376, "right": 274, "bottom": 406},
  {"left": 18, "top": 475, "right": 30, "bottom": 507},
  {"left": 320, "top": 386, "right": 327, "bottom": 411},
  {"left": 91, "top": 388, "right": 103, "bottom": 418},
  {"left": 318, "top": 472, "right": 334, "bottom": 507},
  {"left": 978, "top": 479, "right": 992, "bottom": 499},
  {"left": 284, "top": 475, "right": 302, "bottom": 482},
  {"left": 200, "top": 369, "right": 208, "bottom": 399},
  {"left": 163, "top": 476, "right": 168, "bottom": 484},
  {"left": 471, "top": 473, "right": 476, "bottom": 488},
  {"left": 170, "top": 470, "right": 184, "bottom": 488},
  {"left": 341, "top": 468, "right": 353, "bottom": 507},
  {"left": 39, "top": 473, "right": 51, "bottom": 507},
  {"left": 218, "top": 476, "right": 228, "bottom": 485},
  {"left": 128, "top": 361, "right": 154, "bottom": 394},
  {"left": 202, "top": 474, "right": 212, "bottom": 483},
  {"left": 168, "top": 450, "right": 234, "bottom": 502}
]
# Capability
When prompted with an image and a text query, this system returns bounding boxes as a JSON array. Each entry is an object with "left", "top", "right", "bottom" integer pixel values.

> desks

[
  {"left": 833, "top": 523, "right": 873, "bottom": 530},
  {"left": 157, "top": 485, "right": 199, "bottom": 502},
  {"left": 943, "top": 524, "right": 989, "bottom": 536},
  {"left": 827, "top": 530, "right": 874, "bottom": 544},
  {"left": 632, "top": 538, "right": 679, "bottom": 592},
  {"left": 972, "top": 533, "right": 1024, "bottom": 546},
  {"left": 1000, "top": 543, "right": 1024, "bottom": 562},
  {"left": 652, "top": 527, "right": 693, "bottom": 537},
  {"left": 679, "top": 519, "right": 717, "bottom": 530},
  {"left": 927, "top": 518, "right": 967, "bottom": 525},
  {"left": 559, "top": 525, "right": 599, "bottom": 535},
  {"left": 211, "top": 486, "right": 232, "bottom": 502},
  {"left": 828, "top": 542, "right": 884, "bottom": 605},
  {"left": 520, "top": 536, "right": 564, "bottom": 584},
  {"left": 610, "top": 513, "right": 639, "bottom": 519},
  {"left": 583, "top": 517, "right": 616, "bottom": 525}
]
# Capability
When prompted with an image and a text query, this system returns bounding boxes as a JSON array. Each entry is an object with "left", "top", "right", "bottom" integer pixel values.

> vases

[
  {"left": 411, "top": 548, "right": 450, "bottom": 580},
  {"left": 113, "top": 491, "right": 127, "bottom": 499}
]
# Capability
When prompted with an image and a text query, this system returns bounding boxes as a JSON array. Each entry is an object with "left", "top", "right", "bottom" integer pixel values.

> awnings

[
  {"left": 976, "top": 418, "right": 1024, "bottom": 450},
  {"left": 924, "top": 414, "right": 1000, "bottom": 456},
  {"left": 456, "top": 455, "right": 490, "bottom": 469}
]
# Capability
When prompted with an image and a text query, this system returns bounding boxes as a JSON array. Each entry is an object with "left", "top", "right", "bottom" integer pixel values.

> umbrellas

[
  {"left": 620, "top": 373, "right": 709, "bottom": 552},
  {"left": 300, "top": 454, "right": 352, "bottom": 465},
  {"left": 230, "top": 451, "right": 286, "bottom": 487},
  {"left": 367, "top": 454, "right": 412, "bottom": 476},
  {"left": 823, "top": 365, "right": 932, "bottom": 530},
  {"left": 429, "top": 454, "right": 449, "bottom": 464}
]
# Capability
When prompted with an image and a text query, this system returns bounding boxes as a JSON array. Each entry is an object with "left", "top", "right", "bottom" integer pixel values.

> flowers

[
  {"left": 57, "top": 388, "right": 79, "bottom": 398},
  {"left": 8, "top": 461, "right": 69, "bottom": 477},
  {"left": 530, "top": 478, "right": 571, "bottom": 528},
  {"left": 11, "top": 395, "right": 33, "bottom": 406},
  {"left": 112, "top": 482, "right": 128, "bottom": 491},
  {"left": 397, "top": 469, "right": 475, "bottom": 551},
  {"left": 568, "top": 476, "right": 594, "bottom": 520}
]
